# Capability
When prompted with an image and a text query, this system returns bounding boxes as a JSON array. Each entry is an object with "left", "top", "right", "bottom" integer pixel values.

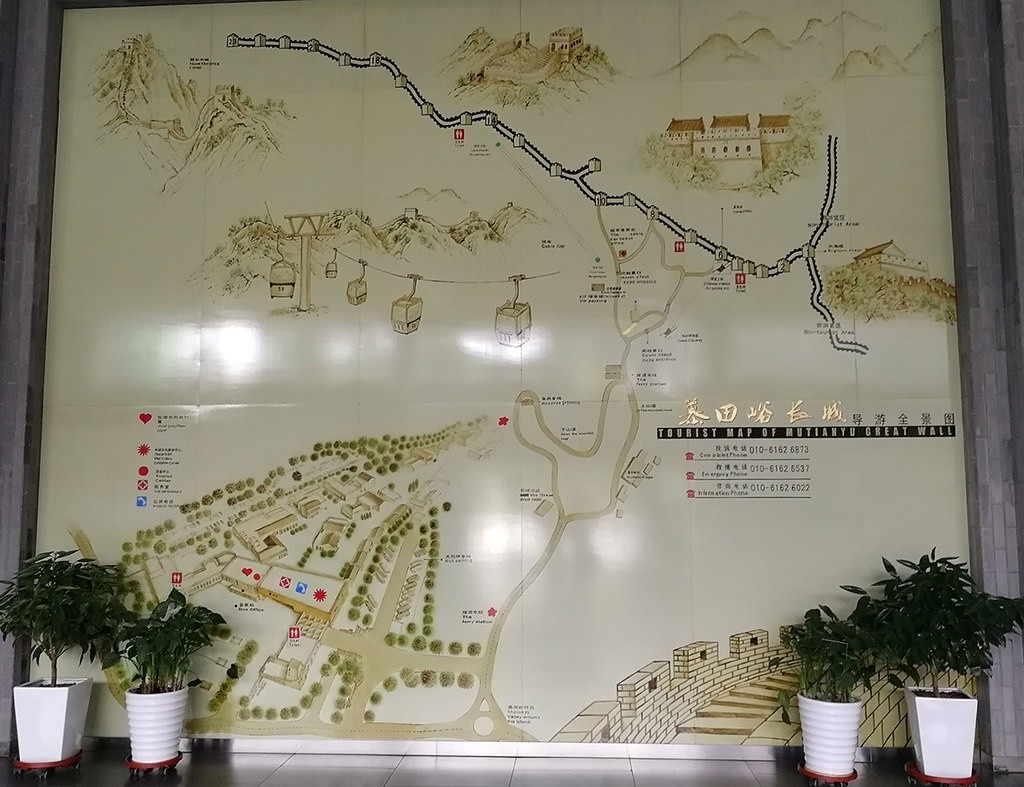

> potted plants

[
  {"left": 103, "top": 589, "right": 230, "bottom": 782},
  {"left": 0, "top": 545, "right": 144, "bottom": 780},
  {"left": 769, "top": 548, "right": 1023, "bottom": 786}
]
